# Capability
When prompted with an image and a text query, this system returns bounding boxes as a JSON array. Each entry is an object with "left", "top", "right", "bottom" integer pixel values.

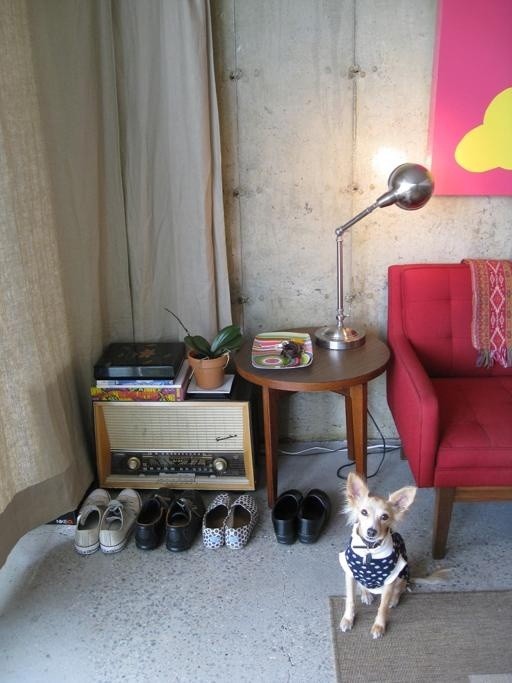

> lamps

[{"left": 314, "top": 164, "right": 434, "bottom": 350}]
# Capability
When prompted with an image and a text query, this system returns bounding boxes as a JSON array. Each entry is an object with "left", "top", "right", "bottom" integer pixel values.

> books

[{"left": 89, "top": 343, "right": 190, "bottom": 402}]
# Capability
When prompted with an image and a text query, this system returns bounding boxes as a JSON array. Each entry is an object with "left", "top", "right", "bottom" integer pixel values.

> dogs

[{"left": 338, "top": 471, "right": 455, "bottom": 638}]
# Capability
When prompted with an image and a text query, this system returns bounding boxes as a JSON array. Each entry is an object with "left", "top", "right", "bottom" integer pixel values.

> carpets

[{"left": 329, "top": 590, "right": 512, "bottom": 683}]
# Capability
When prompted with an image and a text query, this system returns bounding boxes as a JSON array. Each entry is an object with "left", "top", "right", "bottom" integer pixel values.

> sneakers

[
  {"left": 73, "top": 487, "right": 110, "bottom": 556},
  {"left": 223, "top": 494, "right": 259, "bottom": 550},
  {"left": 202, "top": 493, "right": 231, "bottom": 550},
  {"left": 135, "top": 488, "right": 173, "bottom": 549},
  {"left": 98, "top": 488, "right": 143, "bottom": 553},
  {"left": 164, "top": 489, "right": 205, "bottom": 551}
]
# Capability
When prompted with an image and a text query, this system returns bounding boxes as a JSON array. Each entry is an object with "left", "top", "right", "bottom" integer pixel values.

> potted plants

[{"left": 163, "top": 308, "right": 242, "bottom": 389}]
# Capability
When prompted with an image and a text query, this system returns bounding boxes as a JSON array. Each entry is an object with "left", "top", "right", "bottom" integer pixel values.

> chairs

[{"left": 387, "top": 259, "right": 512, "bottom": 559}]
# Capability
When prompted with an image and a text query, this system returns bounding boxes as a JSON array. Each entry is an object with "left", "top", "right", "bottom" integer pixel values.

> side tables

[{"left": 233, "top": 328, "right": 391, "bottom": 509}]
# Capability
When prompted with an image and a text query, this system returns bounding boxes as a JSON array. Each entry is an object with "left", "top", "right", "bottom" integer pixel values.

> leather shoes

[
  {"left": 272, "top": 489, "right": 301, "bottom": 545},
  {"left": 298, "top": 489, "right": 331, "bottom": 544}
]
